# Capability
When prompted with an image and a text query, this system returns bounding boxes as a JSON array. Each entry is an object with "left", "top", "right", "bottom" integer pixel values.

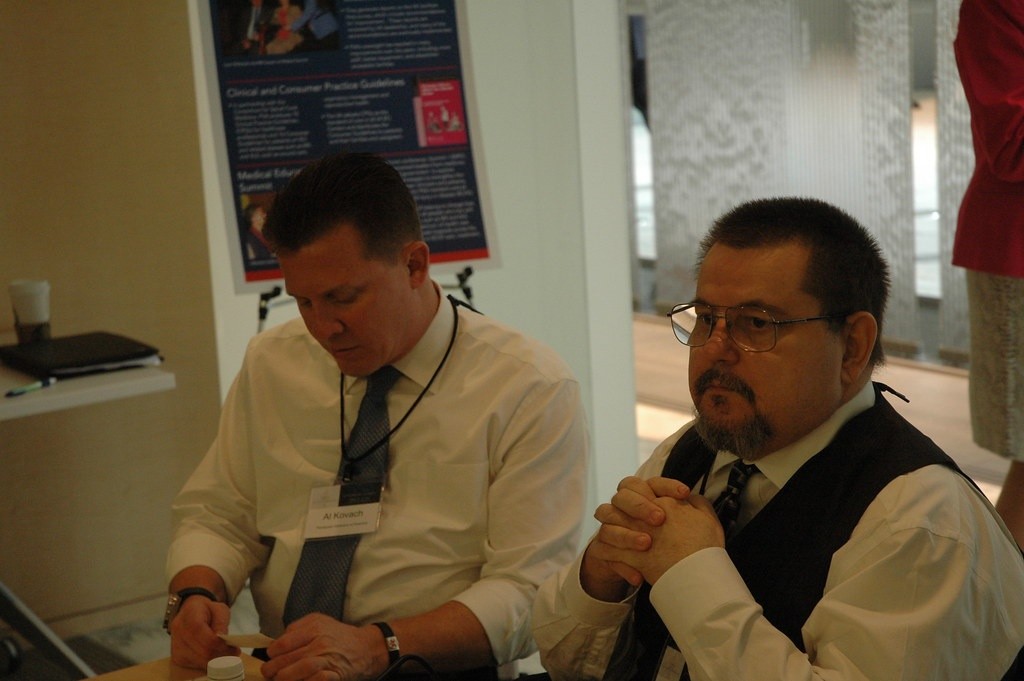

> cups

[{"left": 9, "top": 278, "right": 50, "bottom": 345}]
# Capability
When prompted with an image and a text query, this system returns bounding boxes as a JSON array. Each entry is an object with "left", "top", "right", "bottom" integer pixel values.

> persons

[
  {"left": 531, "top": 198, "right": 1024, "bottom": 681},
  {"left": 951, "top": 0, "right": 1024, "bottom": 556},
  {"left": 161, "top": 155, "right": 587, "bottom": 681}
]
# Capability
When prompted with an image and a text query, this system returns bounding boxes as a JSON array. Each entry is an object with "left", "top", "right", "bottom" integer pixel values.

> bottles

[{"left": 207, "top": 656, "right": 245, "bottom": 681}]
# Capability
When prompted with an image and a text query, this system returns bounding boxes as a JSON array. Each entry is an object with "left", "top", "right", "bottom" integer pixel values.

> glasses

[{"left": 667, "top": 302, "right": 846, "bottom": 352}]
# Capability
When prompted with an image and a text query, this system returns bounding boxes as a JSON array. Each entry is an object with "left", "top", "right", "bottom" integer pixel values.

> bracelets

[{"left": 372, "top": 622, "right": 400, "bottom": 676}]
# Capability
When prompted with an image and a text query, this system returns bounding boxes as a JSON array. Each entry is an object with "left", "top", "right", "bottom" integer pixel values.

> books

[{"left": 0, "top": 330, "right": 166, "bottom": 383}]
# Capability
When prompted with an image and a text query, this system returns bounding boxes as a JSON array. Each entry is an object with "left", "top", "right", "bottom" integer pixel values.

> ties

[
  {"left": 283, "top": 364, "right": 404, "bottom": 631},
  {"left": 711, "top": 458, "right": 761, "bottom": 544}
]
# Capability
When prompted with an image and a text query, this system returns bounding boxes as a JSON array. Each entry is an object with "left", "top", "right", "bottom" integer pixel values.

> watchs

[{"left": 163, "top": 586, "right": 217, "bottom": 634}]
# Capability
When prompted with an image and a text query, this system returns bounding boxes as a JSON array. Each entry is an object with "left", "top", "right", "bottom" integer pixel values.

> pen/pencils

[{"left": 4, "top": 377, "right": 56, "bottom": 398}]
